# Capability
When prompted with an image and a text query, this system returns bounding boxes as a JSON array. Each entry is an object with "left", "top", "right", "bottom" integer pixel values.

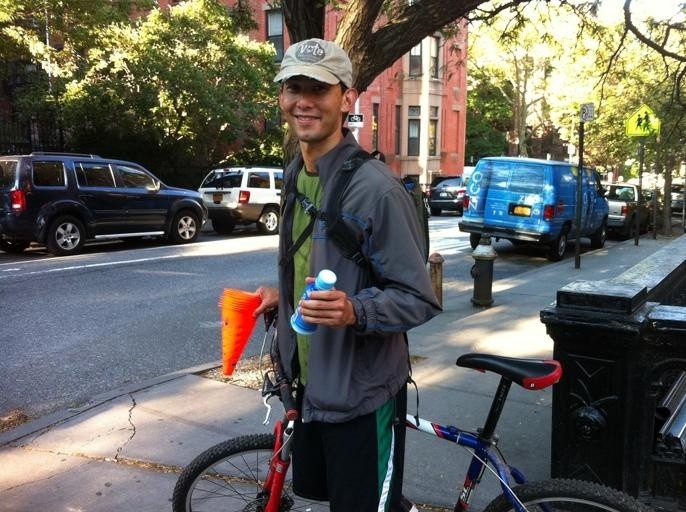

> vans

[
  {"left": 671, "top": 179, "right": 686, "bottom": 213},
  {"left": 457, "top": 153, "right": 613, "bottom": 264}
]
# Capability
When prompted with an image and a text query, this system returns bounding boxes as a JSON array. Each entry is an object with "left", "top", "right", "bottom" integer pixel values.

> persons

[{"left": 251, "top": 38, "right": 445, "bottom": 512}]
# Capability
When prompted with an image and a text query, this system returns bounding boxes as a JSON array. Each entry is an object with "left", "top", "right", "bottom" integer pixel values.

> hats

[{"left": 273, "top": 37, "right": 353, "bottom": 92}]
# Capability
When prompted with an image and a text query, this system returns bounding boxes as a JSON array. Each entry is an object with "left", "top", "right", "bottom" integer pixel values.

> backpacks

[{"left": 282, "top": 151, "right": 429, "bottom": 283}]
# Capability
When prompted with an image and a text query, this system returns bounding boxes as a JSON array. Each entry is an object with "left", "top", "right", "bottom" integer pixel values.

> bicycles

[{"left": 159, "top": 299, "right": 659, "bottom": 511}]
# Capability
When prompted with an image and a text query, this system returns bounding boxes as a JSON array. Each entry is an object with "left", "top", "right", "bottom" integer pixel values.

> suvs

[
  {"left": 196, "top": 160, "right": 283, "bottom": 237},
  {"left": 426, "top": 173, "right": 468, "bottom": 217},
  {"left": 0, "top": 149, "right": 212, "bottom": 259}
]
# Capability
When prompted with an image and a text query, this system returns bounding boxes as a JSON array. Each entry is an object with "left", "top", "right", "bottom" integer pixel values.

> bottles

[{"left": 288, "top": 270, "right": 339, "bottom": 336}]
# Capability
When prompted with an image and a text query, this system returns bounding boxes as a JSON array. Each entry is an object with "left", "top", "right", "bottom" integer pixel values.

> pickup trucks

[{"left": 597, "top": 180, "right": 650, "bottom": 241}]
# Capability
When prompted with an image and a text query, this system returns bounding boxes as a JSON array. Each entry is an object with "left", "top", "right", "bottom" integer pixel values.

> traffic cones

[{"left": 213, "top": 287, "right": 262, "bottom": 382}]
